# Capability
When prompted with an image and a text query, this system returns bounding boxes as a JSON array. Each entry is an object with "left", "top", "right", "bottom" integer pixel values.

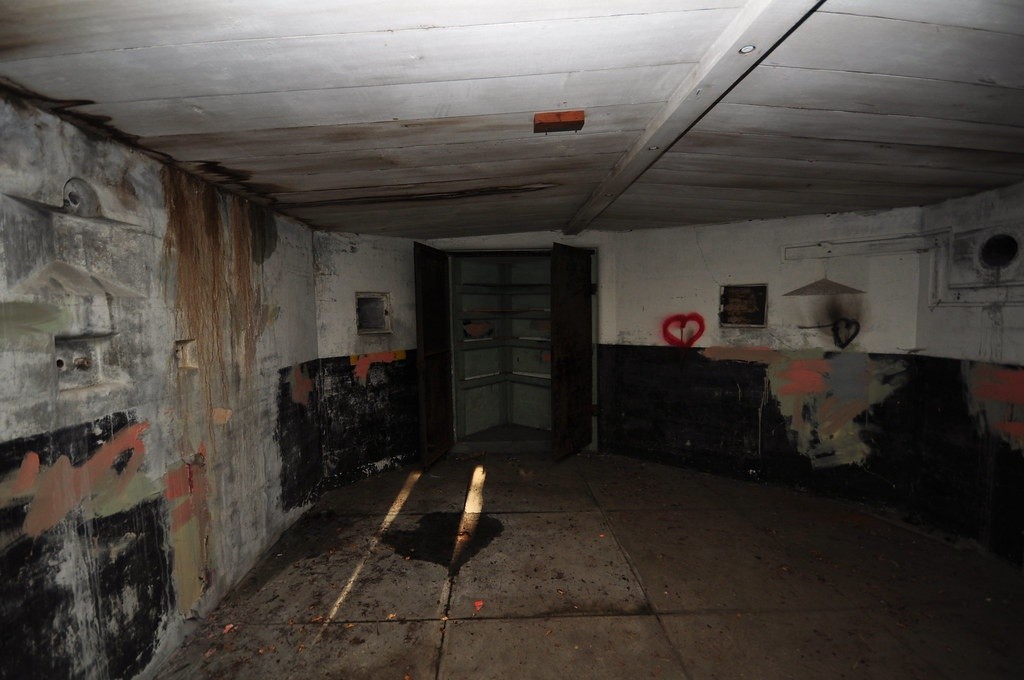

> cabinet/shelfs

[{"left": 411, "top": 239, "right": 595, "bottom": 463}]
